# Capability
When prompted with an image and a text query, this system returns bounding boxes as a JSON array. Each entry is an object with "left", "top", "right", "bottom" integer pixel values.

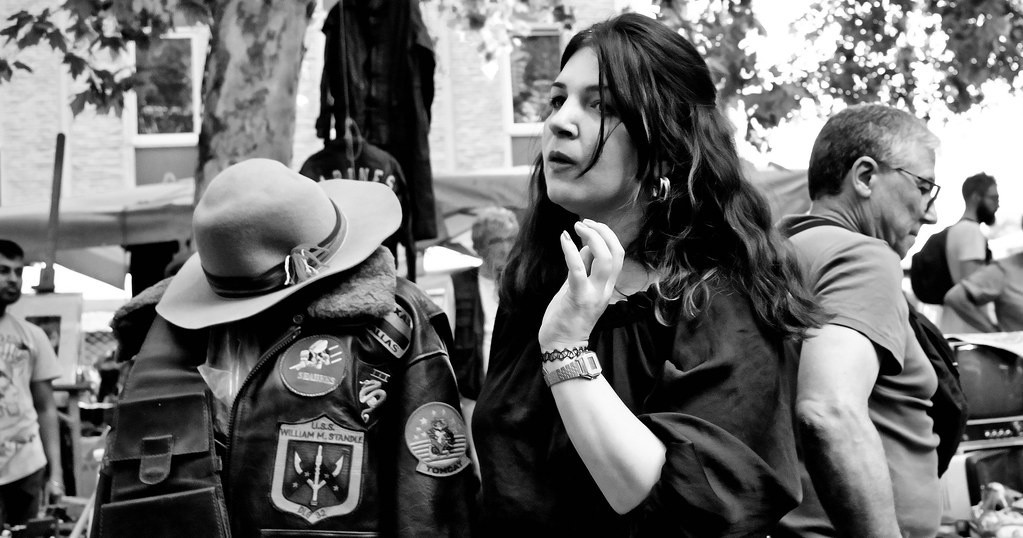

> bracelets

[
  {"left": 542, "top": 352, "right": 602, "bottom": 388},
  {"left": 540, "top": 346, "right": 589, "bottom": 363}
]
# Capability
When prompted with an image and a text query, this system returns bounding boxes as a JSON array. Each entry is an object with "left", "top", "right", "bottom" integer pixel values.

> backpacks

[{"left": 910, "top": 225, "right": 993, "bottom": 306}]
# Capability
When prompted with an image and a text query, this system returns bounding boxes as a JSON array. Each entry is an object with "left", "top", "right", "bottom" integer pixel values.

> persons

[
  {"left": 944, "top": 217, "right": 1023, "bottom": 333},
  {"left": 756, "top": 106, "right": 947, "bottom": 537},
  {"left": 919, "top": 172, "right": 999, "bottom": 333},
  {"left": 1, "top": 139, "right": 522, "bottom": 538},
  {"left": 450, "top": 15, "right": 813, "bottom": 538}
]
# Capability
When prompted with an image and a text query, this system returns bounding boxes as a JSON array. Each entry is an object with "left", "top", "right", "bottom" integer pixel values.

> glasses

[{"left": 893, "top": 168, "right": 941, "bottom": 213}]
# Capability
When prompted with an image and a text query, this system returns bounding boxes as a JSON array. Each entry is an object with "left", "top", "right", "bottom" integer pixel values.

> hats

[{"left": 154, "top": 156, "right": 402, "bottom": 331}]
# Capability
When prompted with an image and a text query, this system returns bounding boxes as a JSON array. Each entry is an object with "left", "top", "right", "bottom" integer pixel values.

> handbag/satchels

[{"left": 904, "top": 294, "right": 968, "bottom": 479}]
